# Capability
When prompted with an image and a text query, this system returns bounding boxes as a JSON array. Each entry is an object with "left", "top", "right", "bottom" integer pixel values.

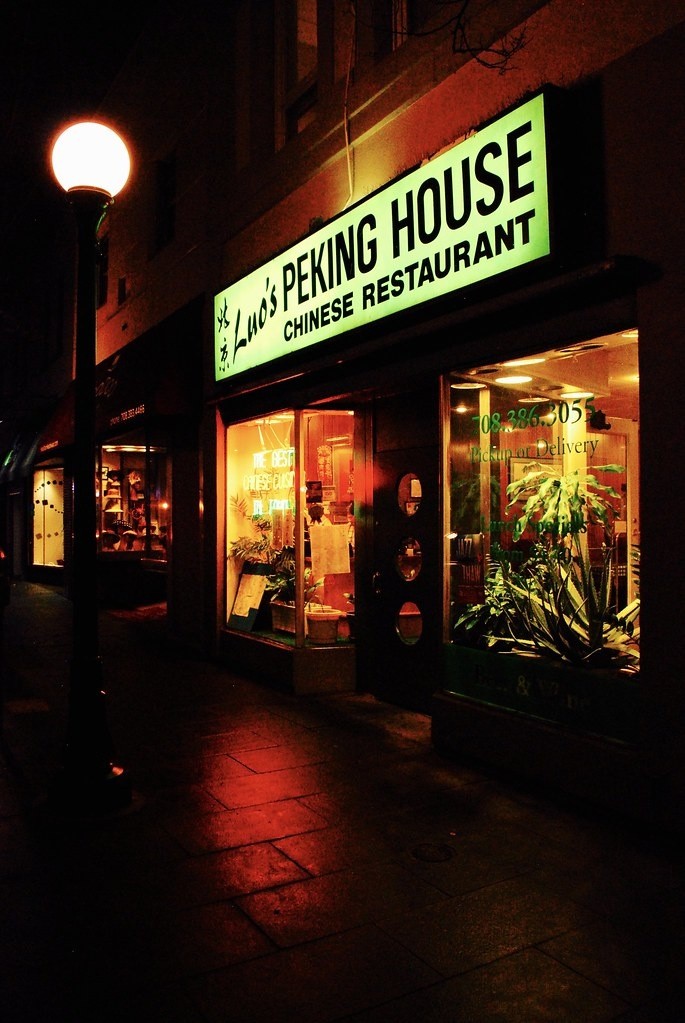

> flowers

[
  {"left": 303, "top": 567, "right": 326, "bottom": 613},
  {"left": 264, "top": 544, "right": 312, "bottom": 598}
]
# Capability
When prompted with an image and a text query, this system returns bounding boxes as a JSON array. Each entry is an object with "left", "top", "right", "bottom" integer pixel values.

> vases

[
  {"left": 306, "top": 608, "right": 340, "bottom": 645},
  {"left": 269, "top": 600, "right": 333, "bottom": 637}
]
